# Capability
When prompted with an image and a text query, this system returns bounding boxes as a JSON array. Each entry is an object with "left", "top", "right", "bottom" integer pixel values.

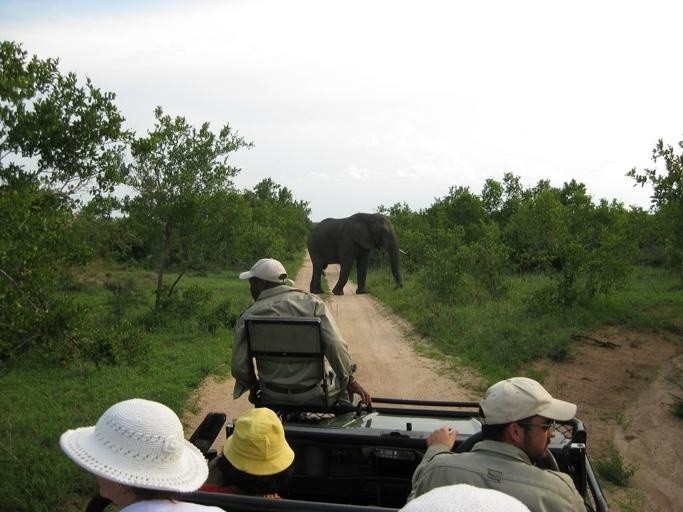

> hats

[
  {"left": 237, "top": 257, "right": 288, "bottom": 285},
  {"left": 220, "top": 406, "right": 296, "bottom": 477},
  {"left": 477, "top": 375, "right": 577, "bottom": 427},
  {"left": 57, "top": 397, "right": 210, "bottom": 494}
]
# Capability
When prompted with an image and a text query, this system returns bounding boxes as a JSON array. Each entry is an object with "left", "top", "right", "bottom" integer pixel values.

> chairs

[{"left": 244, "top": 314, "right": 339, "bottom": 415}]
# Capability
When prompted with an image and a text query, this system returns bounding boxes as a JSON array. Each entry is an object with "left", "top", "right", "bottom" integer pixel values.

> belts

[{"left": 264, "top": 382, "right": 316, "bottom": 395}]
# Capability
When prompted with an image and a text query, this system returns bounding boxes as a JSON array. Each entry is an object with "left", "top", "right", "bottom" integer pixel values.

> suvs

[{"left": 83, "top": 397, "right": 610, "bottom": 512}]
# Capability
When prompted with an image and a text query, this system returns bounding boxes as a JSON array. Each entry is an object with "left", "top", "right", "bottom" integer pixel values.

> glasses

[{"left": 518, "top": 420, "right": 557, "bottom": 430}]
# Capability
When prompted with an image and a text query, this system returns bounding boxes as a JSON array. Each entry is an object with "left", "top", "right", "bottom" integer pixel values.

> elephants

[{"left": 306, "top": 212, "right": 410, "bottom": 295}]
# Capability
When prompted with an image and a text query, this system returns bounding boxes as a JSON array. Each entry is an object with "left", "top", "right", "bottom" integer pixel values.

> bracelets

[{"left": 347, "top": 378, "right": 355, "bottom": 385}]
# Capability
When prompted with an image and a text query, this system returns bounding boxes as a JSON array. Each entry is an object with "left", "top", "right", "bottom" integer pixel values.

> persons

[
  {"left": 408, "top": 376, "right": 589, "bottom": 511},
  {"left": 216, "top": 406, "right": 295, "bottom": 500},
  {"left": 228, "top": 258, "right": 374, "bottom": 406},
  {"left": 59, "top": 397, "right": 224, "bottom": 512}
]
